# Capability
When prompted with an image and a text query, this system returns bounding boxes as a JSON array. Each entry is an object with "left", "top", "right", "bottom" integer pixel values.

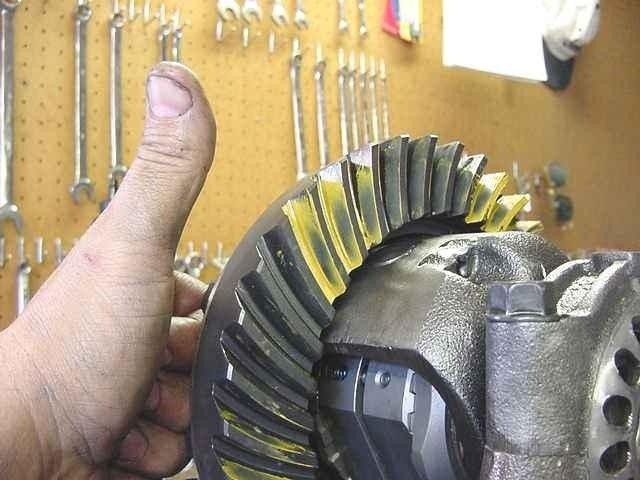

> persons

[{"left": 0, "top": 61, "right": 215, "bottom": 479}]
